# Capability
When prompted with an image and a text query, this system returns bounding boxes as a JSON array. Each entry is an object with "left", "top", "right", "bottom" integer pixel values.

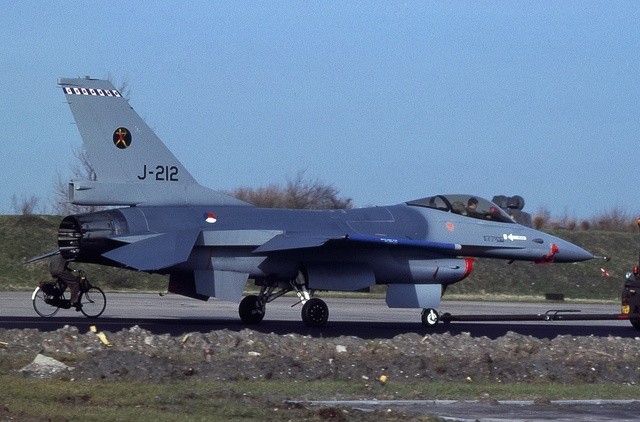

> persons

[
  {"left": 49, "top": 254, "right": 83, "bottom": 307},
  {"left": 466, "top": 198, "right": 491, "bottom": 220}
]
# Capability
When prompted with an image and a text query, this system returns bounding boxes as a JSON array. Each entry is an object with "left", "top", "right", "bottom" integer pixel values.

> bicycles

[{"left": 31, "top": 270, "right": 106, "bottom": 317}]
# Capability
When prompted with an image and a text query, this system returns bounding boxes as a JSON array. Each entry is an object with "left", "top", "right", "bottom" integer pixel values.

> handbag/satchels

[{"left": 78, "top": 270, "right": 89, "bottom": 293}]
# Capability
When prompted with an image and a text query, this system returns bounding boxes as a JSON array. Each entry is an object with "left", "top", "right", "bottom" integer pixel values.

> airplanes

[{"left": 24, "top": 78, "right": 611, "bottom": 330}]
